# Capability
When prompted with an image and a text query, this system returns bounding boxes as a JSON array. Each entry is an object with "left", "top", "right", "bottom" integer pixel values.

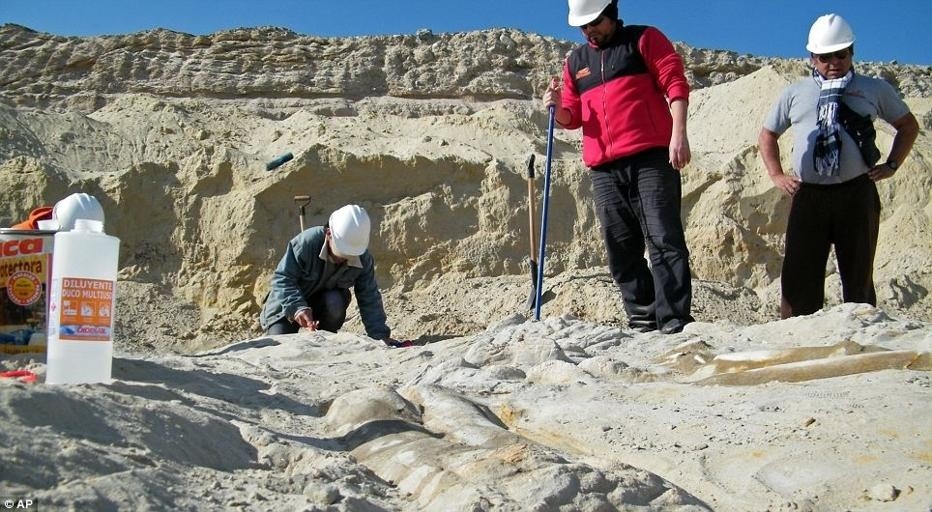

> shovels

[{"left": 525, "top": 154, "right": 538, "bottom": 312}]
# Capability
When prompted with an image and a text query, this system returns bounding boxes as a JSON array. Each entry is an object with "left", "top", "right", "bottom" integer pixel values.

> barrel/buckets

[{"left": 43, "top": 218, "right": 121, "bottom": 387}]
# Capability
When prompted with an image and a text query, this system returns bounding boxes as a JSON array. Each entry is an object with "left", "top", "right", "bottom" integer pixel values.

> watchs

[{"left": 885, "top": 160, "right": 898, "bottom": 171}]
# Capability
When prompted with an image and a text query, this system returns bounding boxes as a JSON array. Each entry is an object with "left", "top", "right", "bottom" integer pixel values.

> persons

[
  {"left": 757, "top": 11, "right": 920, "bottom": 322},
  {"left": 258, "top": 203, "right": 413, "bottom": 349},
  {"left": 542, "top": 0, "right": 702, "bottom": 336}
]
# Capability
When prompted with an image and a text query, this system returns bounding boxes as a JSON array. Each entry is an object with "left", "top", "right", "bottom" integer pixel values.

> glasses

[
  {"left": 580, "top": 18, "right": 604, "bottom": 29},
  {"left": 815, "top": 49, "right": 851, "bottom": 63}
]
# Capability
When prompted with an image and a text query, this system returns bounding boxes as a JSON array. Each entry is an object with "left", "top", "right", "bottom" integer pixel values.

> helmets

[
  {"left": 52, "top": 191, "right": 106, "bottom": 238},
  {"left": 806, "top": 13, "right": 858, "bottom": 55},
  {"left": 329, "top": 203, "right": 371, "bottom": 258},
  {"left": 567, "top": 0, "right": 621, "bottom": 27}
]
